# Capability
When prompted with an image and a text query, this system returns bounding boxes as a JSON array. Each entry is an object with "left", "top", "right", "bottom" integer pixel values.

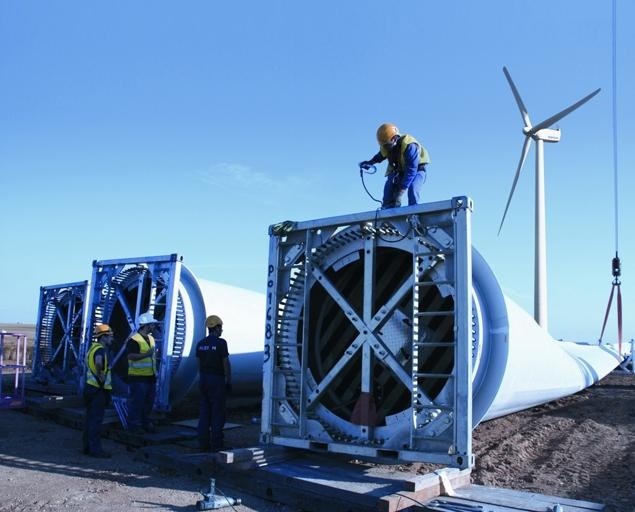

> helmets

[
  {"left": 376, "top": 123, "right": 400, "bottom": 144},
  {"left": 94, "top": 324, "right": 115, "bottom": 337},
  {"left": 137, "top": 312, "right": 158, "bottom": 329},
  {"left": 205, "top": 315, "right": 223, "bottom": 329}
]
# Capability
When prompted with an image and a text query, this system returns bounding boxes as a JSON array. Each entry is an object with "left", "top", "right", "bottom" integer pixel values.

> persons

[
  {"left": 83, "top": 324, "right": 113, "bottom": 458},
  {"left": 360, "top": 124, "right": 430, "bottom": 209},
  {"left": 126, "top": 313, "right": 158, "bottom": 431},
  {"left": 196, "top": 316, "right": 233, "bottom": 451}
]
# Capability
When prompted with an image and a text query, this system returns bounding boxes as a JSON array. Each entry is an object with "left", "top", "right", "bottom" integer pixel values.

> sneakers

[
  {"left": 81, "top": 444, "right": 113, "bottom": 459},
  {"left": 199, "top": 434, "right": 233, "bottom": 450}
]
